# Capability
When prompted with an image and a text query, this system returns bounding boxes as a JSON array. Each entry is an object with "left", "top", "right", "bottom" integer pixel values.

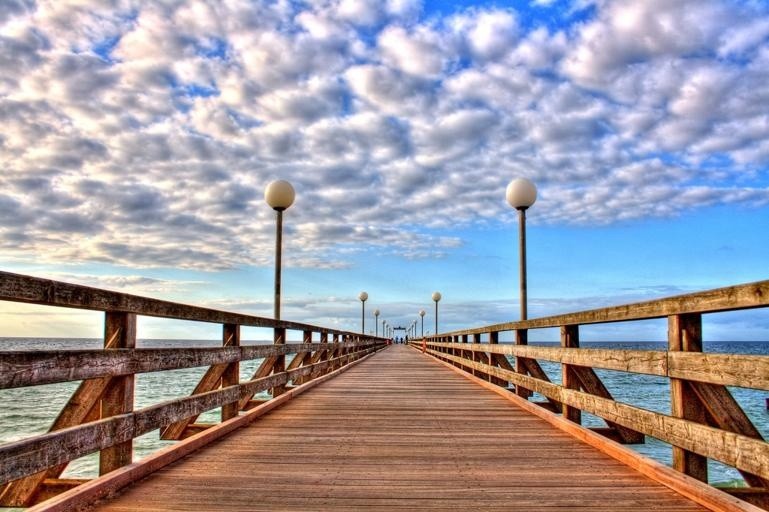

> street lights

[
  {"left": 407, "top": 310, "right": 426, "bottom": 336},
  {"left": 374, "top": 309, "right": 391, "bottom": 338},
  {"left": 506, "top": 179, "right": 537, "bottom": 319},
  {"left": 263, "top": 180, "right": 295, "bottom": 321},
  {"left": 432, "top": 292, "right": 441, "bottom": 335},
  {"left": 359, "top": 292, "right": 368, "bottom": 333}
]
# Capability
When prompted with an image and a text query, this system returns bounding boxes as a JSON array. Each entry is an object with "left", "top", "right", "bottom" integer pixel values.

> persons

[
  {"left": 401, "top": 337, "right": 403, "bottom": 344},
  {"left": 405, "top": 335, "right": 408, "bottom": 345},
  {"left": 396, "top": 336, "right": 398, "bottom": 344}
]
